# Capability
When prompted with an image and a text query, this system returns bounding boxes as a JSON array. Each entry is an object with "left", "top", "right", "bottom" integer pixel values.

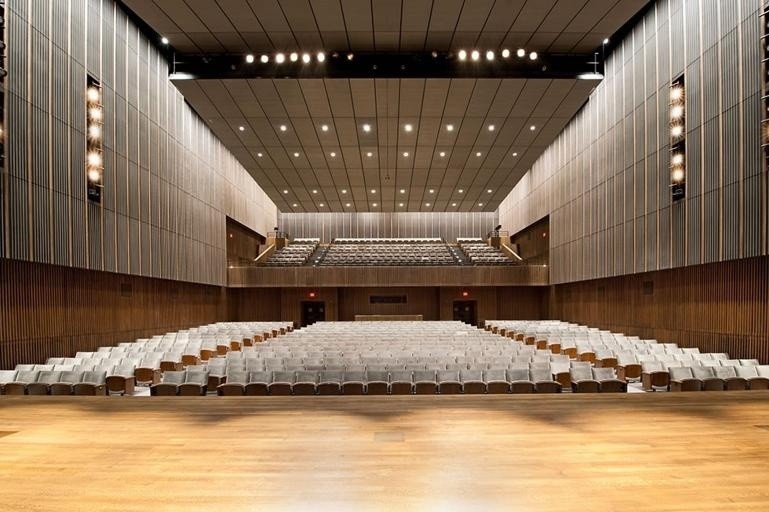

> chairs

[{"left": 253, "top": 227, "right": 519, "bottom": 268}]
